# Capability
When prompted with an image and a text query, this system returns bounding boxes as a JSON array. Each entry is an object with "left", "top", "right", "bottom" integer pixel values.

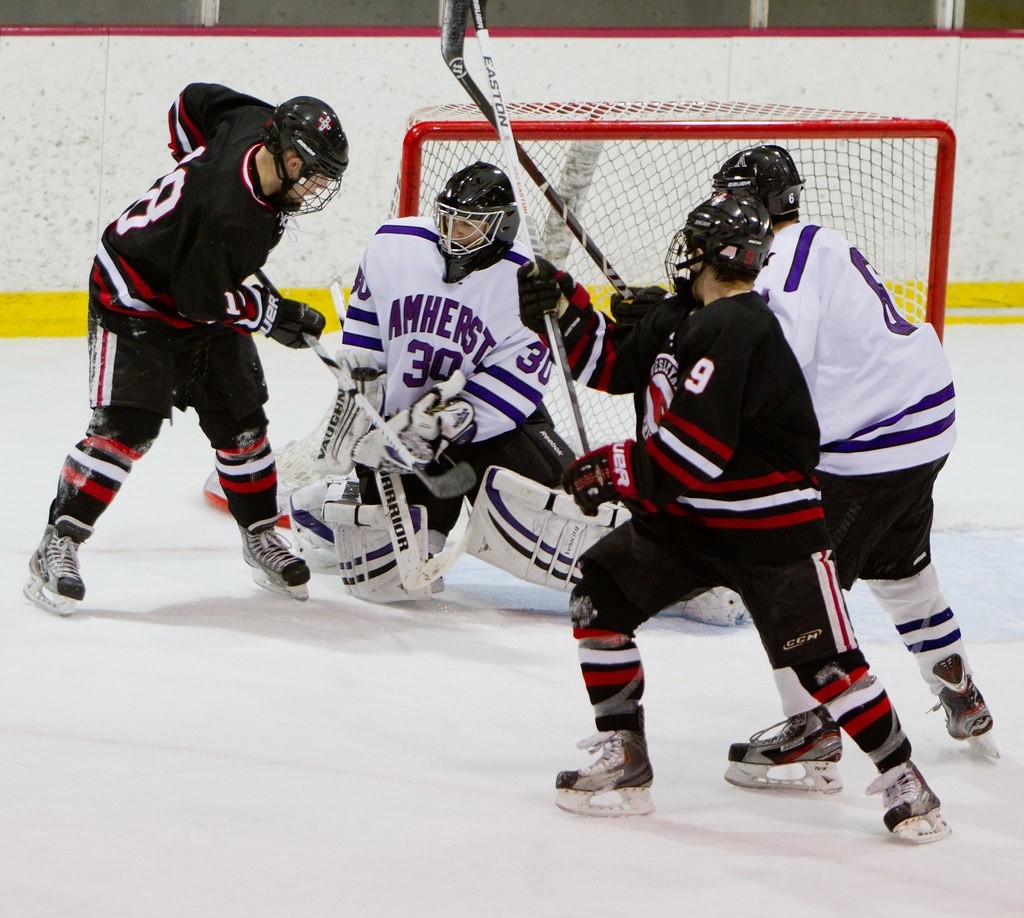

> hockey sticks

[
  {"left": 439, "top": 1, "right": 631, "bottom": 305},
  {"left": 461, "top": 0, "right": 591, "bottom": 459},
  {"left": 255, "top": 269, "right": 478, "bottom": 499},
  {"left": 372, "top": 468, "right": 468, "bottom": 594}
]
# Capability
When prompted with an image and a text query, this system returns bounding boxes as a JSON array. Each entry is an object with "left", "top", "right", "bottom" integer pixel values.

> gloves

[
  {"left": 608, "top": 285, "right": 669, "bottom": 324},
  {"left": 516, "top": 256, "right": 591, "bottom": 348},
  {"left": 561, "top": 439, "right": 649, "bottom": 517},
  {"left": 253, "top": 283, "right": 325, "bottom": 348}
]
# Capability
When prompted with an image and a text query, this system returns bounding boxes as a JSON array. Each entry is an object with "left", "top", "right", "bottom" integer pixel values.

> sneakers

[
  {"left": 866, "top": 759, "right": 951, "bottom": 844},
  {"left": 724, "top": 708, "right": 842, "bottom": 793},
  {"left": 22, "top": 515, "right": 94, "bottom": 616},
  {"left": 237, "top": 507, "right": 311, "bottom": 601},
  {"left": 925, "top": 654, "right": 1002, "bottom": 760},
  {"left": 554, "top": 704, "right": 654, "bottom": 816}
]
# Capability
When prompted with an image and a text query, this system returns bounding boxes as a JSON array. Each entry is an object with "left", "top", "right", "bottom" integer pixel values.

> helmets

[
  {"left": 663, "top": 193, "right": 774, "bottom": 297},
  {"left": 260, "top": 97, "right": 350, "bottom": 217},
  {"left": 712, "top": 145, "right": 804, "bottom": 218},
  {"left": 436, "top": 161, "right": 518, "bottom": 283}
]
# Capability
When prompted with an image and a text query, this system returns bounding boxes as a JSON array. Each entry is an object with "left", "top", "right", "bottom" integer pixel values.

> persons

[
  {"left": 21, "top": 82, "right": 350, "bottom": 617},
  {"left": 282, "top": 161, "right": 751, "bottom": 628},
  {"left": 518, "top": 146, "right": 1001, "bottom": 842}
]
